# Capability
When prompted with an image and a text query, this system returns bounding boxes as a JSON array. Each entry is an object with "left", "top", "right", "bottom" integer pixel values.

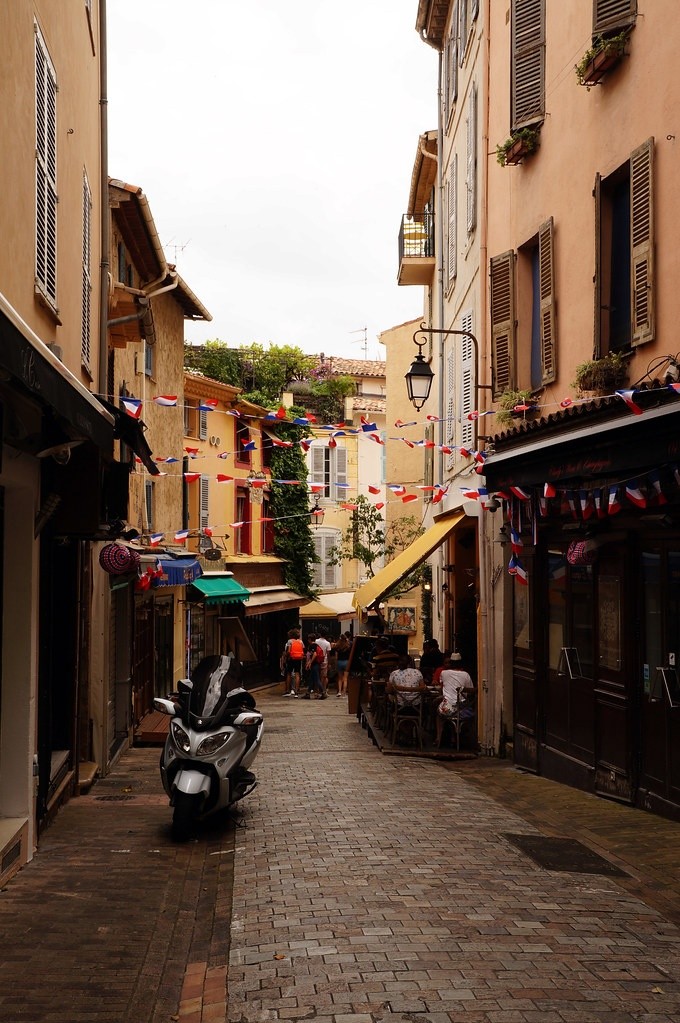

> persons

[
  {"left": 277, "top": 622, "right": 358, "bottom": 699},
  {"left": 365, "top": 633, "right": 475, "bottom": 749}
]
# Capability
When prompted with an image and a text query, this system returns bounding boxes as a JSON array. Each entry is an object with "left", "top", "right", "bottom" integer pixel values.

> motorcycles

[{"left": 152, "top": 654, "right": 266, "bottom": 841}]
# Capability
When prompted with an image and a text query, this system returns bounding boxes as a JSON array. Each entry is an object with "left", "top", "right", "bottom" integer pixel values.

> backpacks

[
  {"left": 289, "top": 638, "right": 304, "bottom": 660},
  {"left": 311, "top": 642, "right": 323, "bottom": 664}
]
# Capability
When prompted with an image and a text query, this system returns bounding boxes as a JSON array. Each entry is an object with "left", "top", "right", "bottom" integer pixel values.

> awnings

[
  {"left": 294, "top": 590, "right": 389, "bottom": 622},
  {"left": 143, "top": 550, "right": 204, "bottom": 587},
  {"left": 351, "top": 497, "right": 481, "bottom": 624},
  {"left": 187, "top": 570, "right": 251, "bottom": 605}
]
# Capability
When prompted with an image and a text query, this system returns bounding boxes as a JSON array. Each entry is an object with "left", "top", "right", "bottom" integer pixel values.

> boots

[
  {"left": 301, "top": 692, "right": 310, "bottom": 699},
  {"left": 319, "top": 691, "right": 329, "bottom": 700}
]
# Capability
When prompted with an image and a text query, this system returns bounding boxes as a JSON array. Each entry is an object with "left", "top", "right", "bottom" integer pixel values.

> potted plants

[
  {"left": 495, "top": 385, "right": 539, "bottom": 427},
  {"left": 495, "top": 127, "right": 540, "bottom": 168},
  {"left": 574, "top": 30, "right": 628, "bottom": 91},
  {"left": 569, "top": 351, "right": 632, "bottom": 398}
]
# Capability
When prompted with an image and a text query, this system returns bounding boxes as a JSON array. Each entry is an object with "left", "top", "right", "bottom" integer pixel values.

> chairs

[{"left": 373, "top": 685, "right": 480, "bottom": 754}]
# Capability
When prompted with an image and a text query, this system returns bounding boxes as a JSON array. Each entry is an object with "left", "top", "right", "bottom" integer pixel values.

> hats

[{"left": 451, "top": 653, "right": 462, "bottom": 661}]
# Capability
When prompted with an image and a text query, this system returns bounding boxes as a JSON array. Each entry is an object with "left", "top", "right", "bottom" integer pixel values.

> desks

[
  {"left": 387, "top": 685, "right": 441, "bottom": 740},
  {"left": 371, "top": 679, "right": 385, "bottom": 717}
]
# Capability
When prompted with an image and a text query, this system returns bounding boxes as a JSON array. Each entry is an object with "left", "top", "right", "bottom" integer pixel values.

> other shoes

[
  {"left": 282, "top": 693, "right": 290, "bottom": 697},
  {"left": 294, "top": 695, "right": 299, "bottom": 698},
  {"left": 336, "top": 693, "right": 342, "bottom": 697}
]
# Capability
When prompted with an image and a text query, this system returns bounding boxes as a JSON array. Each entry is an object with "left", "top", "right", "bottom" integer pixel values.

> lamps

[
  {"left": 305, "top": 491, "right": 325, "bottom": 531},
  {"left": 483, "top": 494, "right": 503, "bottom": 512},
  {"left": 404, "top": 321, "right": 495, "bottom": 462},
  {"left": 442, "top": 564, "right": 453, "bottom": 572},
  {"left": 441, "top": 582, "right": 448, "bottom": 591},
  {"left": 492, "top": 521, "right": 510, "bottom": 547}
]
